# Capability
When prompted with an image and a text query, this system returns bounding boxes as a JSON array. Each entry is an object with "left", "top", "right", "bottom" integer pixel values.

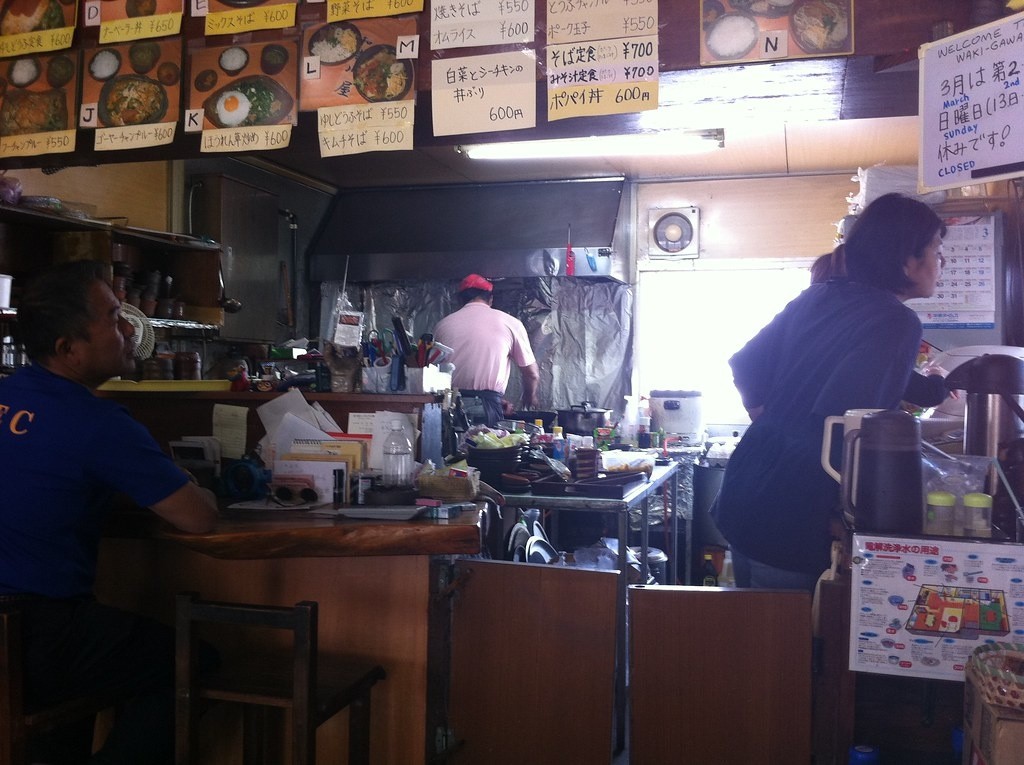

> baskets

[{"left": 970, "top": 642, "right": 1024, "bottom": 713}]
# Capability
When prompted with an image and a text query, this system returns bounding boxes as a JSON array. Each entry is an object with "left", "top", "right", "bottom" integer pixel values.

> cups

[{"left": 261, "top": 45, "right": 289, "bottom": 75}]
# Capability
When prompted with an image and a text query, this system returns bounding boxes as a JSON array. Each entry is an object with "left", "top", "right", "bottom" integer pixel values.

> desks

[
  {"left": 495, "top": 460, "right": 680, "bottom": 750},
  {"left": 99, "top": 502, "right": 483, "bottom": 765},
  {"left": 104, "top": 392, "right": 430, "bottom": 468}
]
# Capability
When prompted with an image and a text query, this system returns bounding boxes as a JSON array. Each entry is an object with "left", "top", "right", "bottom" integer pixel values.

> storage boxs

[{"left": 601, "top": 452, "right": 658, "bottom": 476}]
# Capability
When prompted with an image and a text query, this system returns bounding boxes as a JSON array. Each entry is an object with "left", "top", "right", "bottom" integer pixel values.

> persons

[
  {"left": 0, "top": 261, "right": 232, "bottom": 765},
  {"left": 711, "top": 191, "right": 958, "bottom": 591},
  {"left": 434, "top": 273, "right": 539, "bottom": 427}
]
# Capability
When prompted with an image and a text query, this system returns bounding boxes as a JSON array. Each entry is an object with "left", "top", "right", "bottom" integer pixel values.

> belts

[{"left": 459, "top": 388, "right": 502, "bottom": 404}]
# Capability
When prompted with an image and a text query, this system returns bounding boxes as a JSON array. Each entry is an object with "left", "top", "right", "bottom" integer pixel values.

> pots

[
  {"left": 556, "top": 401, "right": 606, "bottom": 433},
  {"left": 502, "top": 409, "right": 555, "bottom": 432}
]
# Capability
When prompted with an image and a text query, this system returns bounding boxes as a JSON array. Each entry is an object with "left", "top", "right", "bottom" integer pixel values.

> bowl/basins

[
  {"left": 7, "top": 43, "right": 179, "bottom": 87},
  {"left": 705, "top": 11, "right": 759, "bottom": 59},
  {"left": 352, "top": 44, "right": 413, "bottom": 103},
  {"left": 308, "top": 22, "right": 362, "bottom": 65},
  {"left": 702, "top": 0, "right": 725, "bottom": 31},
  {"left": 218, "top": 47, "right": 250, "bottom": 76},
  {"left": 788, "top": 0, "right": 851, "bottom": 54}
]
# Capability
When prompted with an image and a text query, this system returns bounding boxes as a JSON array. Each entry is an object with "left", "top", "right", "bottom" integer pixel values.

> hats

[{"left": 459, "top": 274, "right": 493, "bottom": 291}]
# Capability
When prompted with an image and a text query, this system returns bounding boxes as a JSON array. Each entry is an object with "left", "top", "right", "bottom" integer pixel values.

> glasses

[{"left": 270, "top": 488, "right": 318, "bottom": 507}]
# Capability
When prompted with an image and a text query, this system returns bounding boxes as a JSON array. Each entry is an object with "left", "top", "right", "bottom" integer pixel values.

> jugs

[
  {"left": 839, "top": 409, "right": 923, "bottom": 536},
  {"left": 822, "top": 410, "right": 884, "bottom": 522}
]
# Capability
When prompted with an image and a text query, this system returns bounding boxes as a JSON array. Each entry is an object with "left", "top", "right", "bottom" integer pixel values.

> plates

[
  {"left": 202, "top": 74, "right": 293, "bottom": 128},
  {"left": 194, "top": 70, "right": 218, "bottom": 92},
  {"left": 0, "top": 89, "right": 68, "bottom": 137},
  {"left": 97, "top": 73, "right": 169, "bottom": 127}
]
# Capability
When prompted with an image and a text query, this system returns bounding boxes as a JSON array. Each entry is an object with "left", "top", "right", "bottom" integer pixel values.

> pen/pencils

[{"left": 360, "top": 338, "right": 389, "bottom": 368}]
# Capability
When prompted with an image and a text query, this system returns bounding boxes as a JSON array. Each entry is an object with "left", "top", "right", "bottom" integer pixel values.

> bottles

[
  {"left": 962, "top": 493, "right": 993, "bottom": 538},
  {"left": 699, "top": 555, "right": 718, "bottom": 586},
  {"left": 718, "top": 551, "right": 737, "bottom": 587},
  {"left": 551, "top": 426, "right": 565, "bottom": 460},
  {"left": 535, "top": 419, "right": 545, "bottom": 436},
  {"left": 383, "top": 420, "right": 414, "bottom": 489},
  {"left": 924, "top": 491, "right": 957, "bottom": 537}
]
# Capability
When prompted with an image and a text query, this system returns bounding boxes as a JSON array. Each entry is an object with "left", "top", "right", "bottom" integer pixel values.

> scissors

[{"left": 366, "top": 327, "right": 396, "bottom": 356}]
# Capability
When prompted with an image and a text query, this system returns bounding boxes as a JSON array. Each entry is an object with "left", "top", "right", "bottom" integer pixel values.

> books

[{"left": 275, "top": 437, "right": 366, "bottom": 505}]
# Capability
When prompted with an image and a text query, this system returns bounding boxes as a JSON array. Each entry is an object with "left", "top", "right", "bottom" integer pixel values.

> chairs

[{"left": 173, "top": 599, "right": 386, "bottom": 765}]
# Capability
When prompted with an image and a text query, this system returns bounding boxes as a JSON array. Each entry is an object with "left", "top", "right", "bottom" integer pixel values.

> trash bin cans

[{"left": 629, "top": 545, "right": 669, "bottom": 585}]
